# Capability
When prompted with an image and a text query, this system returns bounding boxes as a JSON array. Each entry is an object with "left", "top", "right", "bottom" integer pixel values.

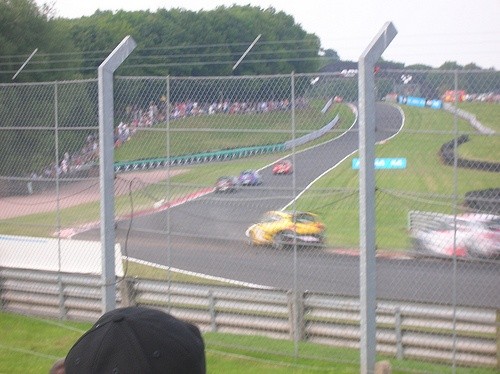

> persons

[
  {"left": 50, "top": 307, "right": 206, "bottom": 374},
  {"left": 28, "top": 92, "right": 318, "bottom": 190}
]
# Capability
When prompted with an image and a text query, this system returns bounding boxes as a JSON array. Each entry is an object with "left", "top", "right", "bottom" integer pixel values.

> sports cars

[
  {"left": 238, "top": 169, "right": 261, "bottom": 186},
  {"left": 408, "top": 210, "right": 499, "bottom": 264},
  {"left": 245, "top": 209, "right": 325, "bottom": 250},
  {"left": 271, "top": 160, "right": 293, "bottom": 176},
  {"left": 216, "top": 175, "right": 238, "bottom": 192}
]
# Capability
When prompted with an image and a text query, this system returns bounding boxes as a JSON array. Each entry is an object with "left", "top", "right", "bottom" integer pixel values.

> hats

[{"left": 65, "top": 306, "right": 208, "bottom": 374}]
